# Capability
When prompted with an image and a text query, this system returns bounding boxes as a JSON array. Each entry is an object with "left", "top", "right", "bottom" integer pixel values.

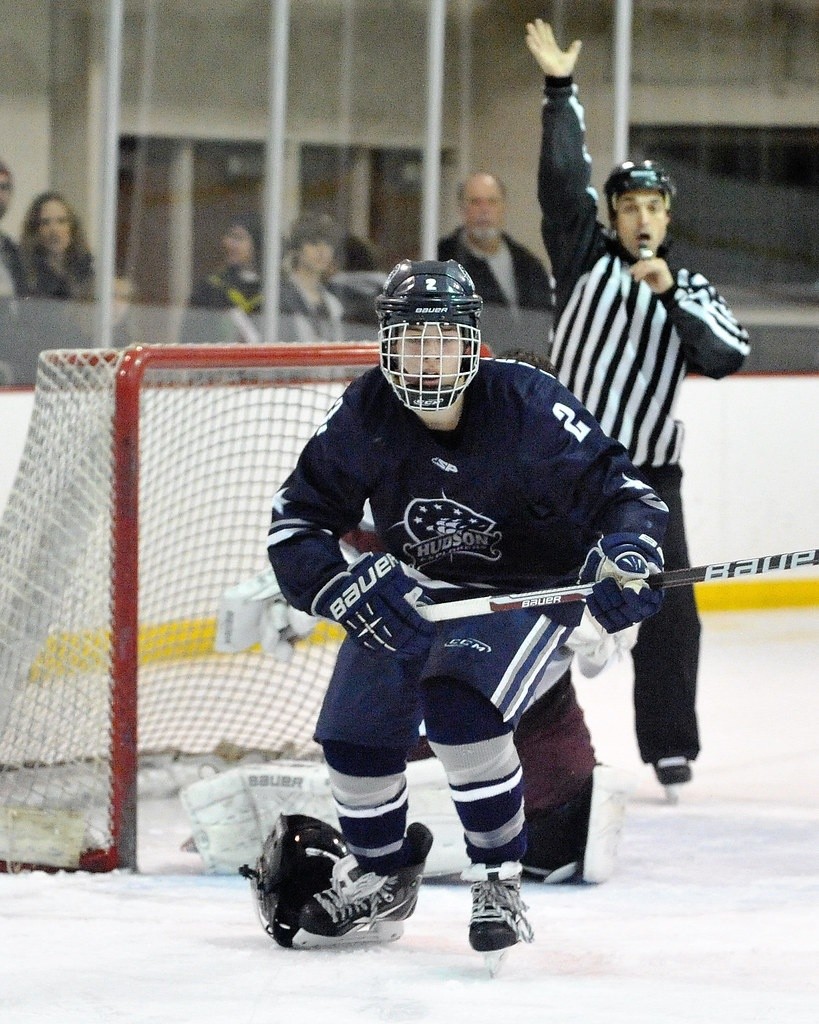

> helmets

[
  {"left": 374, "top": 259, "right": 483, "bottom": 413},
  {"left": 604, "top": 160, "right": 676, "bottom": 219}
]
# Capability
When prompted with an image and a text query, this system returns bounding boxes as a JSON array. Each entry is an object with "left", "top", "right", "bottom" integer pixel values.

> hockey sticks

[{"left": 413, "top": 548, "right": 819, "bottom": 624}]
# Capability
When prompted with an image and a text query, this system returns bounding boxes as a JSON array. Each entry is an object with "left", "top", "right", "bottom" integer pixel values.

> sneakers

[
  {"left": 652, "top": 754, "right": 691, "bottom": 804},
  {"left": 460, "top": 860, "right": 535, "bottom": 979},
  {"left": 291, "top": 822, "right": 434, "bottom": 950}
]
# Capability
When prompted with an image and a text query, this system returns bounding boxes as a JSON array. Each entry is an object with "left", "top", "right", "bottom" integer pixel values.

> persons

[
  {"left": 436, "top": 171, "right": 551, "bottom": 311},
  {"left": 524, "top": 18, "right": 750, "bottom": 806},
  {"left": 184, "top": 211, "right": 373, "bottom": 344},
  {"left": 0, "top": 161, "right": 145, "bottom": 383},
  {"left": 269, "top": 257, "right": 670, "bottom": 979}
]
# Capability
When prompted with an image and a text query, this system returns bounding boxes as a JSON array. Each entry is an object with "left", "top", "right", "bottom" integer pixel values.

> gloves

[
  {"left": 576, "top": 531, "right": 665, "bottom": 634},
  {"left": 311, "top": 552, "right": 443, "bottom": 660}
]
